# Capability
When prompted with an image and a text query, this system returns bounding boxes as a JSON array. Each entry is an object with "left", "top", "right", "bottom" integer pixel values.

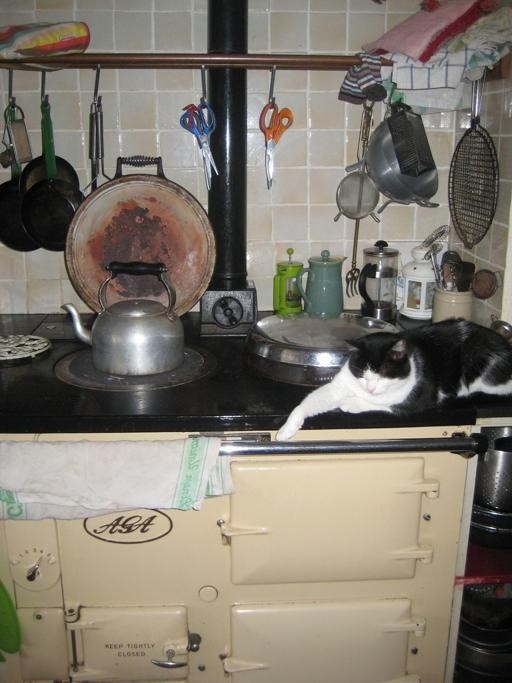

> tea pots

[
  {"left": 61, "top": 260, "right": 183, "bottom": 377},
  {"left": 298, "top": 249, "right": 348, "bottom": 313}
]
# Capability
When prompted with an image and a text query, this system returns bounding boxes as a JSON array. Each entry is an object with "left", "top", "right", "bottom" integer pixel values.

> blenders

[
  {"left": 273, "top": 246, "right": 303, "bottom": 315},
  {"left": 357, "top": 240, "right": 402, "bottom": 322}
]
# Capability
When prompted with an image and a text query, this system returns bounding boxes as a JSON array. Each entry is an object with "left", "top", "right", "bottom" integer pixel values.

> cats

[{"left": 275, "top": 316, "right": 512, "bottom": 442}]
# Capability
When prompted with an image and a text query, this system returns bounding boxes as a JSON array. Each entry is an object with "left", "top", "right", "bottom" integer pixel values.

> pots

[
  {"left": 0, "top": 100, "right": 86, "bottom": 253},
  {"left": 365, "top": 103, "right": 440, "bottom": 213},
  {"left": 63, "top": 154, "right": 217, "bottom": 318}
]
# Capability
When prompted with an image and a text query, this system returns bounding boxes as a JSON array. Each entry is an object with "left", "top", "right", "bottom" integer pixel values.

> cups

[{"left": 432, "top": 286, "right": 473, "bottom": 324}]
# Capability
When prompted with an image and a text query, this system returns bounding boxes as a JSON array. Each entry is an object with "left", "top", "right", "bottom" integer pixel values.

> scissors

[
  {"left": 259, "top": 103, "right": 293, "bottom": 190},
  {"left": 184, "top": 103, "right": 216, "bottom": 192},
  {"left": 180, "top": 103, "right": 219, "bottom": 178}
]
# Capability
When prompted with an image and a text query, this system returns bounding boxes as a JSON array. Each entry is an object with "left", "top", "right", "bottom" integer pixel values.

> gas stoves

[{"left": 0, "top": 312, "right": 484, "bottom": 683}]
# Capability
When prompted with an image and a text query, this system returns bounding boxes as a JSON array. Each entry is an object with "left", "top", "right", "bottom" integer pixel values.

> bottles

[{"left": 399, "top": 245, "right": 436, "bottom": 320}]
